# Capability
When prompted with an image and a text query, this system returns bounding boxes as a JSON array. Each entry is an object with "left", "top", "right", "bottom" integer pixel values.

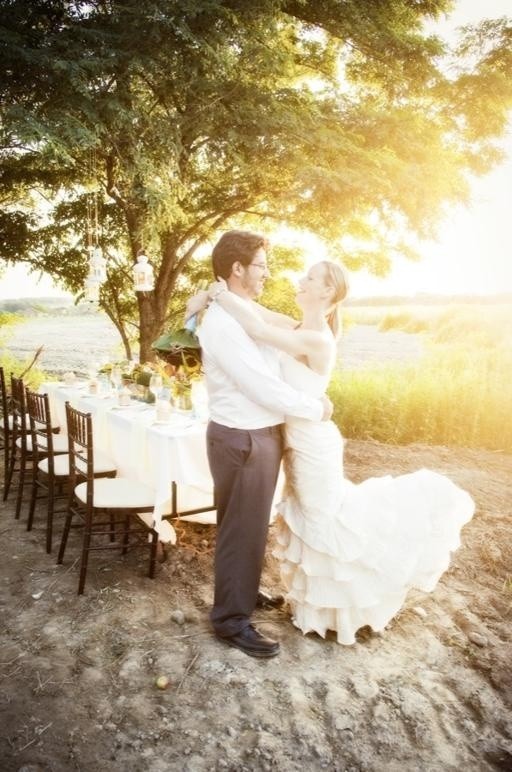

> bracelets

[{"left": 214, "top": 288, "right": 225, "bottom": 298}]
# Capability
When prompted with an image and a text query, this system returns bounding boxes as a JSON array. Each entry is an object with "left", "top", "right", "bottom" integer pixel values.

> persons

[
  {"left": 193, "top": 229, "right": 336, "bottom": 661},
  {"left": 180, "top": 258, "right": 355, "bottom": 648}
]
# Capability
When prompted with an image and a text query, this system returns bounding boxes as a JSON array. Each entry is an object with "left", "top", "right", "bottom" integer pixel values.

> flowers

[{"left": 150, "top": 277, "right": 213, "bottom": 378}]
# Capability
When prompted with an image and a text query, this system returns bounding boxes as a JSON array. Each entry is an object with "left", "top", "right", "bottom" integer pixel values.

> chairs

[{"left": 0, "top": 366, "right": 165, "bottom": 593}]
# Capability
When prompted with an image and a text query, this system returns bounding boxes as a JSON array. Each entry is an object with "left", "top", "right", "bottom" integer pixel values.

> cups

[{"left": 63, "top": 363, "right": 210, "bottom": 426}]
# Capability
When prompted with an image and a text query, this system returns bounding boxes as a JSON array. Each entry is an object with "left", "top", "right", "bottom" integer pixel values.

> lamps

[{"left": 84, "top": 140, "right": 153, "bottom": 304}]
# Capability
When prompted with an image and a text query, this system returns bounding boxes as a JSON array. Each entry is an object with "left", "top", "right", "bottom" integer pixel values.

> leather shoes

[
  {"left": 216, "top": 623, "right": 280, "bottom": 658},
  {"left": 256, "top": 590, "right": 285, "bottom": 608}
]
